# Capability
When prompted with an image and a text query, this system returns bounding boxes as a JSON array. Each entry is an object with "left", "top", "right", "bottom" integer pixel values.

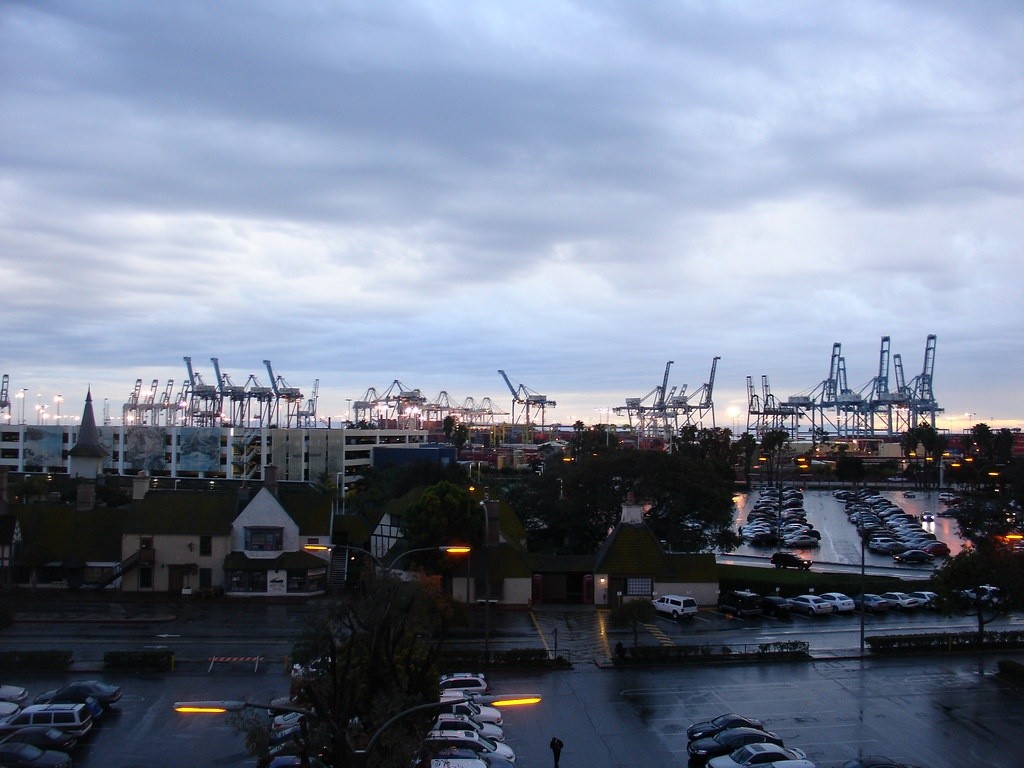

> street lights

[
  {"left": 346, "top": 398, "right": 352, "bottom": 419},
  {"left": 22, "top": 388, "right": 29, "bottom": 425},
  {"left": 478, "top": 498, "right": 493, "bottom": 662},
  {"left": 301, "top": 542, "right": 474, "bottom": 587},
  {"left": 57, "top": 394, "right": 62, "bottom": 425},
  {"left": 172, "top": 691, "right": 544, "bottom": 768}
]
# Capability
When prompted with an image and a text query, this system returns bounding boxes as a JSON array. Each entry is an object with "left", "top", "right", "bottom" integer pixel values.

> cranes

[{"left": 102, "top": 397, "right": 112, "bottom": 426}]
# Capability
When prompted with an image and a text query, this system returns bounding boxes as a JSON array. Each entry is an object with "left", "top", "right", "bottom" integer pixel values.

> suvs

[
  {"left": 953, "top": 585, "right": 1005, "bottom": 608},
  {"left": 771, "top": 551, "right": 813, "bottom": 570},
  {"left": 650, "top": 594, "right": 699, "bottom": 620}
]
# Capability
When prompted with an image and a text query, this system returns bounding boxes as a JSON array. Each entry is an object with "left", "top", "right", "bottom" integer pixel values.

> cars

[
  {"left": 785, "top": 595, "right": 833, "bottom": 616},
  {"left": 877, "top": 592, "right": 920, "bottom": 610},
  {"left": 716, "top": 591, "right": 794, "bottom": 620},
  {"left": 413, "top": 672, "right": 517, "bottom": 768},
  {"left": 686, "top": 713, "right": 764, "bottom": 741},
  {"left": 852, "top": 593, "right": 891, "bottom": 613},
  {"left": 738, "top": 486, "right": 961, "bottom": 565},
  {"left": 254, "top": 655, "right": 336, "bottom": 768},
  {"left": 704, "top": 742, "right": 816, "bottom": 768},
  {"left": 0, "top": 684, "right": 103, "bottom": 768},
  {"left": 834, "top": 756, "right": 917, "bottom": 768},
  {"left": 34, "top": 680, "right": 123, "bottom": 704},
  {"left": 907, "top": 591, "right": 948, "bottom": 610},
  {"left": 736, "top": 760, "right": 816, "bottom": 768},
  {"left": 687, "top": 727, "right": 785, "bottom": 762},
  {"left": 817, "top": 592, "right": 856, "bottom": 613}
]
